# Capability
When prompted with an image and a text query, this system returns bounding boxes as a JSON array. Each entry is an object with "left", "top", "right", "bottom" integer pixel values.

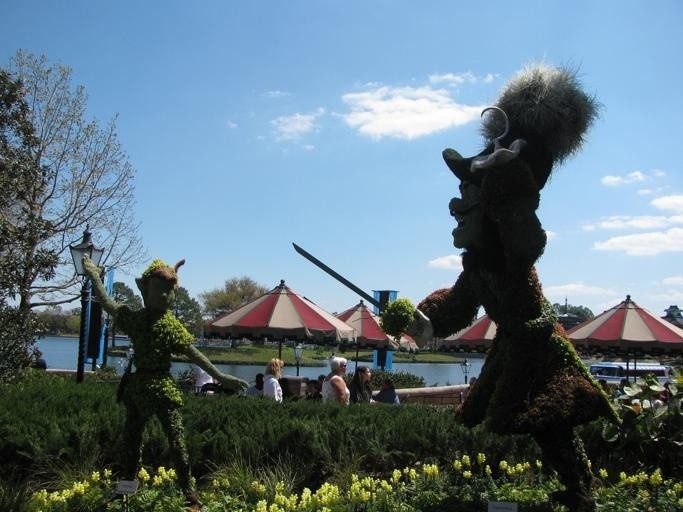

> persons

[
  {"left": 263, "top": 358, "right": 284, "bottom": 403},
  {"left": 303, "top": 357, "right": 399, "bottom": 406},
  {"left": 245, "top": 373, "right": 264, "bottom": 399},
  {"left": 188, "top": 363, "right": 217, "bottom": 395},
  {"left": 80, "top": 253, "right": 250, "bottom": 512}
]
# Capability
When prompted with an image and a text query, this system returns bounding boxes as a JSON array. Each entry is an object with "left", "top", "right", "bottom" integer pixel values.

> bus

[{"left": 589, "top": 364, "right": 672, "bottom": 388}]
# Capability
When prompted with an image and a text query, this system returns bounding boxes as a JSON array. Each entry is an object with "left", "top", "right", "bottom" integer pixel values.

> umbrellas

[
  {"left": 431, "top": 315, "right": 498, "bottom": 344},
  {"left": 333, "top": 299, "right": 420, "bottom": 367},
  {"left": 564, "top": 294, "right": 683, "bottom": 380},
  {"left": 210, "top": 279, "right": 354, "bottom": 355}
]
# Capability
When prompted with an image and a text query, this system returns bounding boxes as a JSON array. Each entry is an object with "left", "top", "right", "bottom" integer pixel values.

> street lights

[
  {"left": 293, "top": 343, "right": 305, "bottom": 375},
  {"left": 460, "top": 354, "right": 473, "bottom": 383},
  {"left": 68, "top": 224, "right": 104, "bottom": 384}
]
